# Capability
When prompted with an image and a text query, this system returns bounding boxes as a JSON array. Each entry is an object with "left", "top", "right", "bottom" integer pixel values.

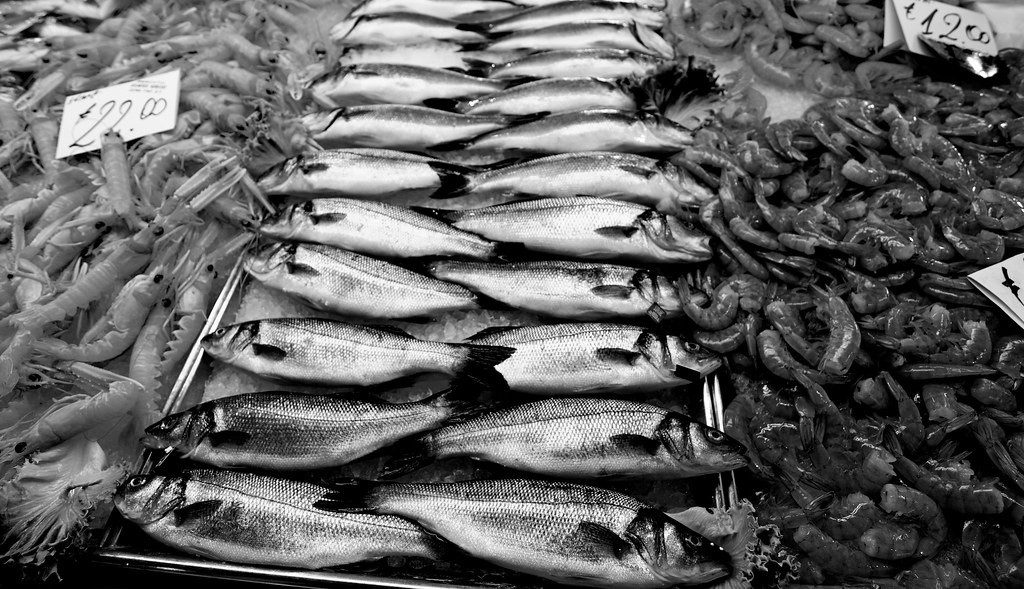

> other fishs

[{"left": 115, "top": 0, "right": 754, "bottom": 589}]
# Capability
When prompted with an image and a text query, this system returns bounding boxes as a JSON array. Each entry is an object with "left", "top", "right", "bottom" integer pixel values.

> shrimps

[
  {"left": 668, "top": 47, "right": 1024, "bottom": 589},
  {"left": 664, "top": 0, "right": 915, "bottom": 99},
  {"left": 0, "top": 0, "right": 319, "bottom": 465}
]
